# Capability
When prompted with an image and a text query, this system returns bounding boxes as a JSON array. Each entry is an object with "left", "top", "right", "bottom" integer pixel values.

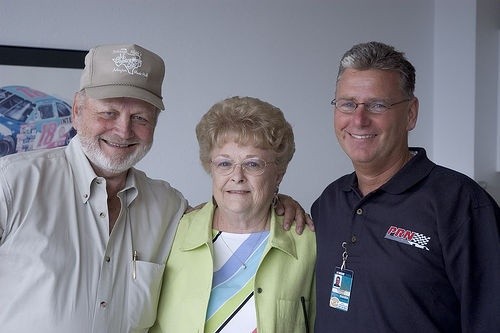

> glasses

[
  {"left": 329, "top": 97, "right": 415, "bottom": 114},
  {"left": 210, "top": 159, "right": 278, "bottom": 176}
]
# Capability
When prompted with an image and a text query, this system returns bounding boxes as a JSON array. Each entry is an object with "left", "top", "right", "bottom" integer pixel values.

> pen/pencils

[{"left": 132, "top": 250, "right": 139, "bottom": 279}]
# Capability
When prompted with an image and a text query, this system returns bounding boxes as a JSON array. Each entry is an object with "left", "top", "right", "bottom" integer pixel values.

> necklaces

[{"left": 217, "top": 206, "right": 266, "bottom": 269}]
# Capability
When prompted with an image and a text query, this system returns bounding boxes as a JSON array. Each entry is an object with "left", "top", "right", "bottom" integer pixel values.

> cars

[{"left": 0, "top": 85, "right": 77, "bottom": 156}]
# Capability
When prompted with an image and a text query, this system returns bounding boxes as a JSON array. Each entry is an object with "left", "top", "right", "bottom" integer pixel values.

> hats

[{"left": 80, "top": 43, "right": 165, "bottom": 110}]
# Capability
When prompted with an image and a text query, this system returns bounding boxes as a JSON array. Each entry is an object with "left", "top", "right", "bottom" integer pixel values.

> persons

[
  {"left": 0, "top": 43, "right": 314, "bottom": 333},
  {"left": 309, "top": 41, "right": 500, "bottom": 333},
  {"left": 334, "top": 277, "right": 340, "bottom": 287},
  {"left": 148, "top": 96, "right": 318, "bottom": 333}
]
